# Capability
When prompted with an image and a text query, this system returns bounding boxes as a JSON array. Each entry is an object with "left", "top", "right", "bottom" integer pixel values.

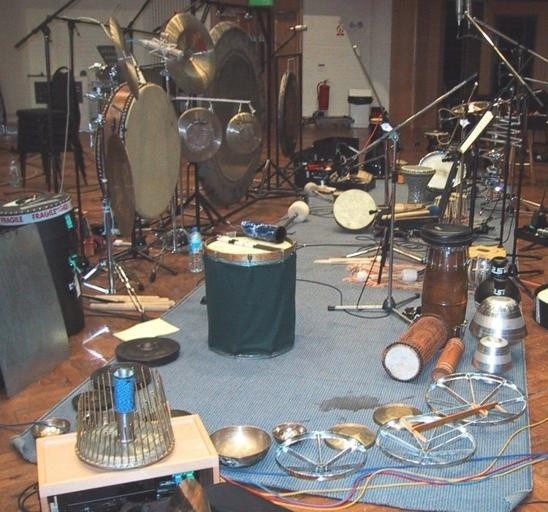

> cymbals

[
  {"left": 227, "top": 113, "right": 262, "bottom": 154},
  {"left": 277, "top": 70, "right": 299, "bottom": 157},
  {"left": 184, "top": 20, "right": 265, "bottom": 205},
  {"left": 177, "top": 108, "right": 222, "bottom": 164},
  {"left": 374, "top": 403, "right": 425, "bottom": 430},
  {"left": 324, "top": 424, "right": 376, "bottom": 451},
  {"left": 106, "top": 133, "right": 135, "bottom": 237},
  {"left": 450, "top": 100, "right": 492, "bottom": 114},
  {"left": 162, "top": 12, "right": 216, "bottom": 94},
  {"left": 109, "top": 15, "right": 140, "bottom": 100}
]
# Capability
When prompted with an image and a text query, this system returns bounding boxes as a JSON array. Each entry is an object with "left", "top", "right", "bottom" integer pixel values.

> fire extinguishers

[{"left": 317, "top": 79, "right": 330, "bottom": 111}]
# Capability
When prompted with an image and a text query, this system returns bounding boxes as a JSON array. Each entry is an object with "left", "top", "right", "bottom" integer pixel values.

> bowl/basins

[
  {"left": 32, "top": 418, "right": 71, "bottom": 438},
  {"left": 469, "top": 296, "right": 529, "bottom": 375},
  {"left": 209, "top": 422, "right": 308, "bottom": 467}
]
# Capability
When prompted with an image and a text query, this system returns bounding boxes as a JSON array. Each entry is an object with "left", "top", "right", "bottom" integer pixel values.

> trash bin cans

[{"left": 348, "top": 88, "right": 373, "bottom": 128}]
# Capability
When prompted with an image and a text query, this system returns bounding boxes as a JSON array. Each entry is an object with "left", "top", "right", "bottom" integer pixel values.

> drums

[
  {"left": 424, "top": 130, "right": 449, "bottom": 151},
  {"left": 417, "top": 150, "right": 467, "bottom": 198},
  {"left": 203, "top": 234, "right": 296, "bottom": 357},
  {"left": 95, "top": 82, "right": 180, "bottom": 219},
  {"left": 402, "top": 165, "right": 436, "bottom": 203},
  {"left": 421, "top": 223, "right": 474, "bottom": 338},
  {"left": 332, "top": 190, "right": 375, "bottom": 234},
  {"left": 381, "top": 312, "right": 453, "bottom": 382},
  {"left": 0, "top": 193, "right": 72, "bottom": 226}
]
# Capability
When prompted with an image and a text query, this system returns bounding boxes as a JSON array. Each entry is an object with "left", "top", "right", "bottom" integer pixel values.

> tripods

[
  {"left": 245, "top": 8, "right": 301, "bottom": 195},
  {"left": 165, "top": 94, "right": 229, "bottom": 237},
  {"left": 81, "top": 88, "right": 146, "bottom": 321},
  {"left": 110, "top": 216, "right": 178, "bottom": 277},
  {"left": 345, "top": 43, "right": 426, "bottom": 265},
  {"left": 327, "top": 74, "right": 482, "bottom": 326}
]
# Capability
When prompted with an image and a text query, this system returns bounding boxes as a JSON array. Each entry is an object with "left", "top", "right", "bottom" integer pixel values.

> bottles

[
  {"left": 188, "top": 226, "right": 206, "bottom": 273},
  {"left": 8, "top": 161, "right": 24, "bottom": 189},
  {"left": 241, "top": 219, "right": 287, "bottom": 243}
]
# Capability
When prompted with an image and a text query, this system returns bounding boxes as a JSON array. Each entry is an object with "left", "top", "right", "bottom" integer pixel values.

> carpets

[{"left": 6, "top": 180, "right": 534, "bottom": 510}]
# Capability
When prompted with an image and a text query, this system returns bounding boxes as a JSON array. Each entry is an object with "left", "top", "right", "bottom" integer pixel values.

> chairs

[{"left": 15, "top": 67, "right": 87, "bottom": 195}]
